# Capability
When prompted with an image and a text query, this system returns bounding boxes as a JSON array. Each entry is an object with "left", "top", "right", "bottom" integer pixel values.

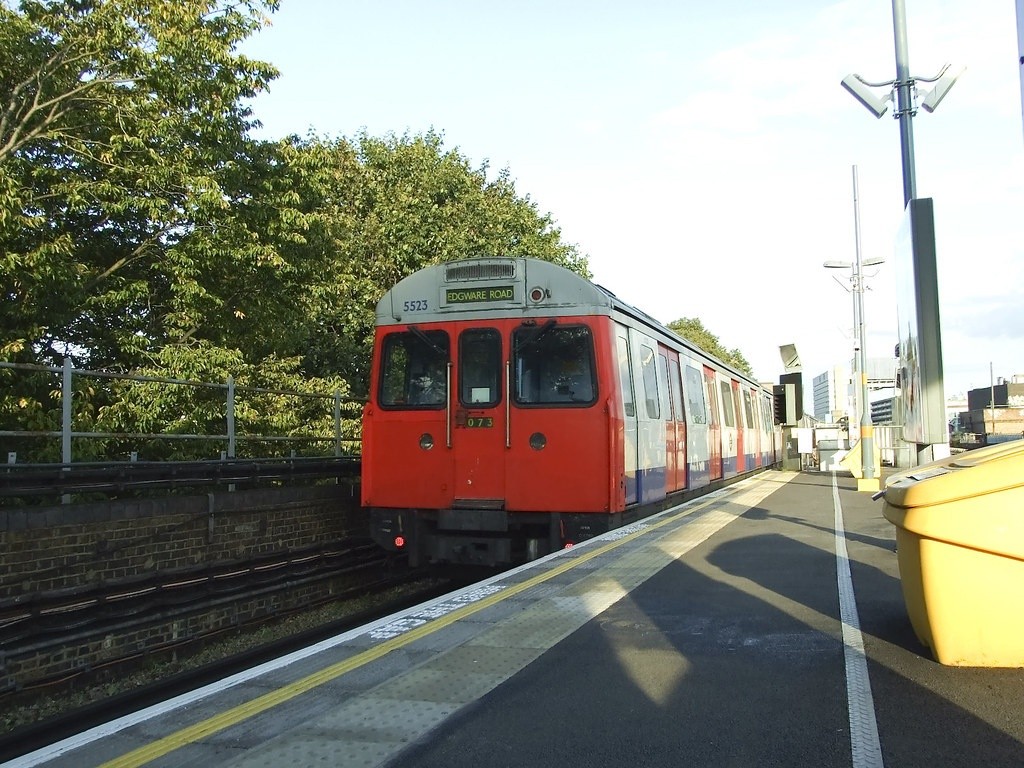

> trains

[{"left": 360, "top": 256, "right": 822, "bottom": 578}]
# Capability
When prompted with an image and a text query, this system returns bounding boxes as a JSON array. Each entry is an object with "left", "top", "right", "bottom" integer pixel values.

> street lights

[{"left": 823, "top": 256, "right": 886, "bottom": 375}]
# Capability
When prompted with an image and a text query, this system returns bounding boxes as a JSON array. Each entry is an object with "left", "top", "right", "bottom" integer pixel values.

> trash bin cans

[
  {"left": 839, "top": 427, "right": 882, "bottom": 478},
  {"left": 875, "top": 438, "right": 1024, "bottom": 669}
]
